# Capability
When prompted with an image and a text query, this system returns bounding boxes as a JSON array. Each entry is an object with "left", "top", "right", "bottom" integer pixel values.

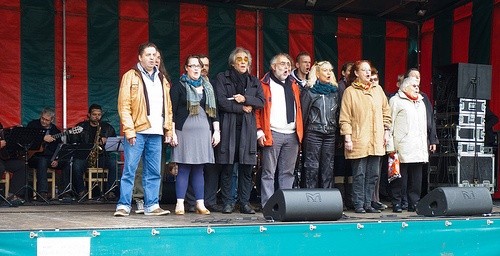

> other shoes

[
  {"left": 393, "top": 203, "right": 402, "bottom": 213},
  {"left": 355, "top": 207, "right": 365, "bottom": 213},
  {"left": 402, "top": 203, "right": 408, "bottom": 210},
  {"left": 222, "top": 204, "right": 232, "bottom": 213},
  {"left": 408, "top": 203, "right": 416, "bottom": 211},
  {"left": 59, "top": 192, "right": 71, "bottom": 202},
  {"left": 106, "top": 191, "right": 118, "bottom": 200},
  {"left": 240, "top": 204, "right": 255, "bottom": 214},
  {"left": 76, "top": 190, "right": 87, "bottom": 201},
  {"left": 35, "top": 192, "right": 48, "bottom": 202},
  {"left": 187, "top": 205, "right": 194, "bottom": 212},
  {"left": 4, "top": 193, "right": 26, "bottom": 206},
  {"left": 144, "top": 208, "right": 170, "bottom": 216},
  {"left": 365, "top": 206, "right": 374, "bottom": 212},
  {"left": 371, "top": 201, "right": 388, "bottom": 210},
  {"left": 114, "top": 209, "right": 129, "bottom": 217},
  {"left": 208, "top": 204, "right": 223, "bottom": 211}
]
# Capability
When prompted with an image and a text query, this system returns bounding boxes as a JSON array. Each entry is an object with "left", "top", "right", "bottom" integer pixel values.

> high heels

[
  {"left": 194, "top": 204, "right": 210, "bottom": 215},
  {"left": 175, "top": 204, "right": 184, "bottom": 215}
]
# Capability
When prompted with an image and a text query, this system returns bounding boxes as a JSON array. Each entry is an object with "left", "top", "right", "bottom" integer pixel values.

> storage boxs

[
  {"left": 434, "top": 112, "right": 486, "bottom": 127},
  {"left": 434, "top": 96, "right": 486, "bottom": 114},
  {"left": 436, "top": 140, "right": 484, "bottom": 154},
  {"left": 436, "top": 126, "right": 484, "bottom": 142},
  {"left": 428, "top": 153, "right": 494, "bottom": 196}
]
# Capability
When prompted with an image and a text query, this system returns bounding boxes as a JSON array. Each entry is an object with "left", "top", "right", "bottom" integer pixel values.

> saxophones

[{"left": 87, "top": 120, "right": 102, "bottom": 168}]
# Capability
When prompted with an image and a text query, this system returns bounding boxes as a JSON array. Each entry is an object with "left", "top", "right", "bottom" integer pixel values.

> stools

[
  {"left": 0, "top": 171, "right": 14, "bottom": 200},
  {"left": 83, "top": 167, "right": 108, "bottom": 200},
  {"left": 33, "top": 168, "right": 55, "bottom": 202}
]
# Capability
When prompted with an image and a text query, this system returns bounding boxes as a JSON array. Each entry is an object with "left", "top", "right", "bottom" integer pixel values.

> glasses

[
  {"left": 370, "top": 78, "right": 379, "bottom": 82},
  {"left": 188, "top": 65, "right": 201, "bottom": 69},
  {"left": 410, "top": 84, "right": 420, "bottom": 88}
]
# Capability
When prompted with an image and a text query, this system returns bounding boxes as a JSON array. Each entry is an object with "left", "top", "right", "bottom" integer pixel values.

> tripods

[
  {"left": 98, "top": 136, "right": 127, "bottom": 200},
  {"left": 7, "top": 127, "right": 50, "bottom": 205},
  {"left": 56, "top": 143, "right": 94, "bottom": 201}
]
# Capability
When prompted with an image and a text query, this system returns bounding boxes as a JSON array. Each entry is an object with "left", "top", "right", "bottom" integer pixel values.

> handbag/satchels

[{"left": 387, "top": 154, "right": 401, "bottom": 183}]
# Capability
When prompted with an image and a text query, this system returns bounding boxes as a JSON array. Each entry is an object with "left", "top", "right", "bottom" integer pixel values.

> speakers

[
  {"left": 436, "top": 63, "right": 492, "bottom": 99},
  {"left": 262, "top": 189, "right": 343, "bottom": 220},
  {"left": 415, "top": 187, "right": 494, "bottom": 217}
]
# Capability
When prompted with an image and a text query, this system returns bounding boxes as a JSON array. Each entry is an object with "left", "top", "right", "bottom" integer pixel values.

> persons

[
  {"left": 187, "top": 55, "right": 226, "bottom": 214},
  {"left": 254, "top": 53, "right": 305, "bottom": 213},
  {"left": 332, "top": 63, "right": 355, "bottom": 211},
  {"left": 132, "top": 47, "right": 173, "bottom": 214},
  {"left": 168, "top": 55, "right": 211, "bottom": 216},
  {"left": 215, "top": 48, "right": 265, "bottom": 214},
  {"left": 112, "top": 43, "right": 173, "bottom": 216},
  {"left": 339, "top": 60, "right": 438, "bottom": 214},
  {"left": 288, "top": 52, "right": 312, "bottom": 95},
  {"left": 65, "top": 103, "right": 121, "bottom": 201},
  {"left": 24, "top": 107, "right": 73, "bottom": 203},
  {"left": 297, "top": 60, "right": 340, "bottom": 193},
  {"left": 0, "top": 123, "right": 32, "bottom": 202}
]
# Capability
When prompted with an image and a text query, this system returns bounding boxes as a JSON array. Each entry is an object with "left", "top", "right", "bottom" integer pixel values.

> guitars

[{"left": 21, "top": 125, "right": 83, "bottom": 160}]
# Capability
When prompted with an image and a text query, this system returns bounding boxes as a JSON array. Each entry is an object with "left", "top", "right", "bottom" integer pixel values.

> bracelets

[
  {"left": 345, "top": 141, "right": 352, "bottom": 143},
  {"left": 214, "top": 129, "right": 221, "bottom": 132}
]
